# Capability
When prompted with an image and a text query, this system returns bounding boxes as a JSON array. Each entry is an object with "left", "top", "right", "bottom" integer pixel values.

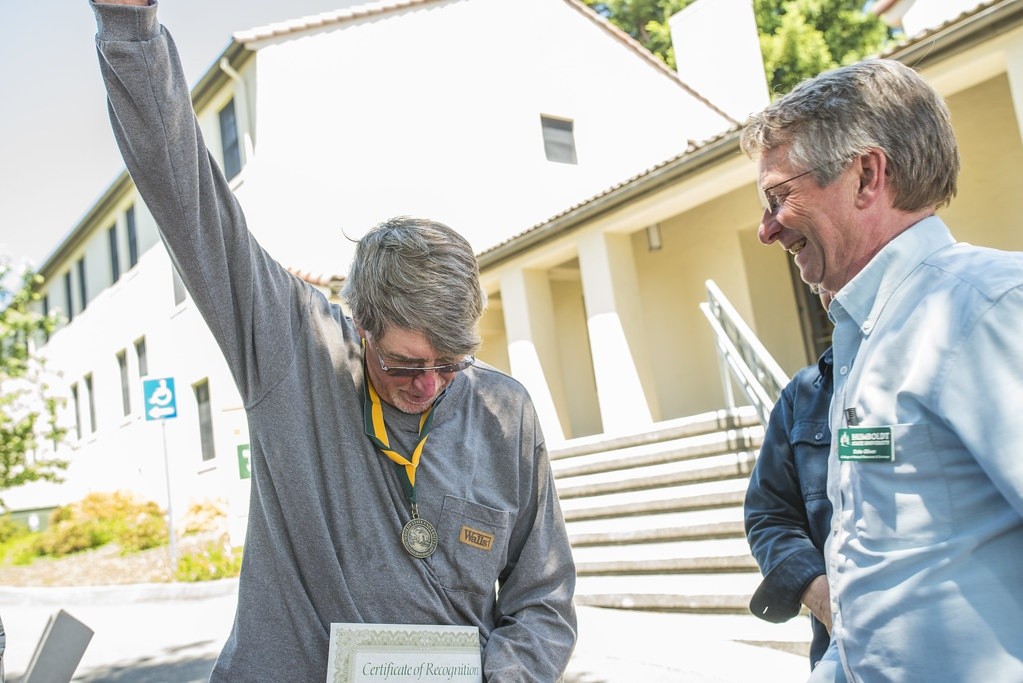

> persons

[
  {"left": 739, "top": 58, "right": 1023, "bottom": 683},
  {"left": 92, "top": 2, "right": 578, "bottom": 683},
  {"left": 744, "top": 284, "right": 837, "bottom": 672}
]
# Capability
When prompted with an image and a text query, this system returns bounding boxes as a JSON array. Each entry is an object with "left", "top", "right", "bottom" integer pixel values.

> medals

[{"left": 401, "top": 518, "right": 437, "bottom": 559}]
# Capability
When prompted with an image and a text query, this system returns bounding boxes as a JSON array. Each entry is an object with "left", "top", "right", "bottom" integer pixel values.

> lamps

[{"left": 646, "top": 223, "right": 663, "bottom": 252}]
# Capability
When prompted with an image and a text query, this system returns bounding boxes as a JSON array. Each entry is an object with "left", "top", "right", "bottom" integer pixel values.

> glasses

[
  {"left": 365, "top": 332, "right": 474, "bottom": 378},
  {"left": 754, "top": 148, "right": 894, "bottom": 214}
]
